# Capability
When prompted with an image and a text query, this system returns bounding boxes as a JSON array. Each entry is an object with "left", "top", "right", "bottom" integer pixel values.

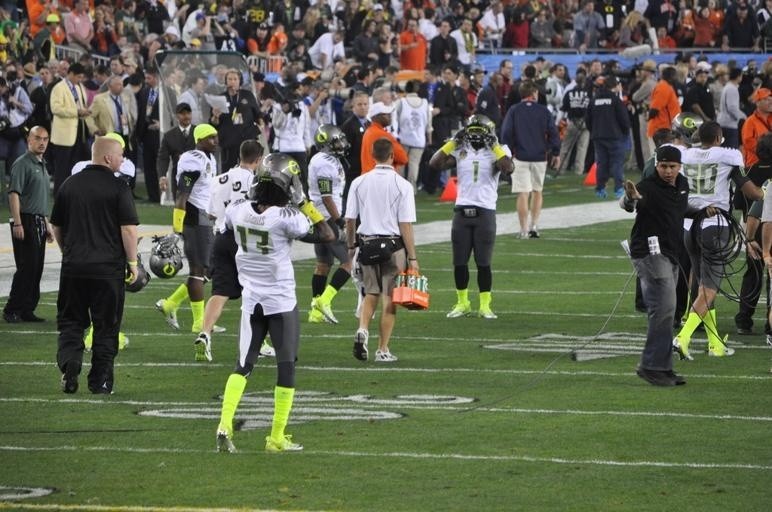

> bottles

[
  {"left": 395, "top": 273, "right": 405, "bottom": 288},
  {"left": 417, "top": 278, "right": 427, "bottom": 292},
  {"left": 406, "top": 274, "right": 417, "bottom": 288}
]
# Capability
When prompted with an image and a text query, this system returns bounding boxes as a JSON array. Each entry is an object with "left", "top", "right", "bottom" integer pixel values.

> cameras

[
  {"left": 271, "top": 82, "right": 301, "bottom": 117},
  {"left": 324, "top": 88, "right": 355, "bottom": 102}
]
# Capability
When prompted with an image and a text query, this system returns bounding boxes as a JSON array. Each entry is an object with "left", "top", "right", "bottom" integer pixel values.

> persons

[
  {"left": 48, "top": 138, "right": 138, "bottom": 395},
  {"left": 545, "top": 62, "right": 570, "bottom": 113},
  {"left": 627, "top": 65, "right": 645, "bottom": 171},
  {"left": 251, "top": 71, "right": 275, "bottom": 149},
  {"left": 155, "top": 124, "right": 227, "bottom": 333},
  {"left": 673, "top": 54, "right": 683, "bottom": 70},
  {"left": 716, "top": 68, "right": 748, "bottom": 149},
  {"left": 588, "top": 58, "right": 602, "bottom": 81},
  {"left": 287, "top": 83, "right": 303, "bottom": 101},
  {"left": 760, "top": 61, "right": 772, "bottom": 89},
  {"left": 681, "top": 70, "right": 715, "bottom": 124},
  {"left": 584, "top": 77, "right": 631, "bottom": 200},
  {"left": 49, "top": 64, "right": 91, "bottom": 169},
  {"left": 658, "top": 120, "right": 772, "bottom": 361},
  {"left": 368, "top": 87, "right": 397, "bottom": 135},
  {"left": 767, "top": 56, "right": 772, "bottom": 63},
  {"left": 216, "top": 150, "right": 335, "bottom": 455},
  {"left": 177, "top": 70, "right": 208, "bottom": 125},
  {"left": 674, "top": 63, "right": 688, "bottom": 96},
  {"left": 733, "top": 132, "right": 772, "bottom": 335},
  {"left": 458, "top": 69, "right": 476, "bottom": 113},
  {"left": 194, "top": 140, "right": 277, "bottom": 362},
  {"left": 0, "top": 0, "right": 477, "bottom": 71},
  {"left": 743, "top": 58, "right": 760, "bottom": 77},
  {"left": 760, "top": 184, "right": 772, "bottom": 335},
  {"left": 727, "top": 59, "right": 739, "bottom": 72},
  {"left": 341, "top": 91, "right": 371, "bottom": 184},
  {"left": 470, "top": 70, "right": 485, "bottom": 93},
  {"left": 208, "top": 71, "right": 262, "bottom": 150},
  {"left": 475, "top": 72, "right": 503, "bottom": 126},
  {"left": 497, "top": 59, "right": 515, "bottom": 102},
  {"left": 162, "top": 69, "right": 178, "bottom": 115},
  {"left": 506, "top": 65, "right": 548, "bottom": 108},
  {"left": 125, "top": 73, "right": 143, "bottom": 134},
  {"left": 71, "top": 132, "right": 135, "bottom": 353},
  {"left": 271, "top": 89, "right": 306, "bottom": 151},
  {"left": 300, "top": 78, "right": 328, "bottom": 136},
  {"left": 135, "top": 68, "right": 174, "bottom": 204},
  {"left": 708, "top": 67, "right": 729, "bottom": 117},
  {"left": 657, "top": 62, "right": 669, "bottom": 75},
  {"left": 670, "top": 110, "right": 704, "bottom": 148},
  {"left": 417, "top": 67, "right": 439, "bottom": 102},
  {"left": 697, "top": 56, "right": 709, "bottom": 63},
  {"left": 390, "top": 78, "right": 434, "bottom": 196},
  {"left": 531, "top": 56, "right": 546, "bottom": 80},
  {"left": 372, "top": 66, "right": 399, "bottom": 89},
  {"left": 480, "top": 0, "right": 772, "bottom": 51},
  {"left": 575, "top": 60, "right": 588, "bottom": 70},
  {"left": 734, "top": 179, "right": 772, "bottom": 335},
  {"left": 84, "top": 76, "right": 141, "bottom": 201},
  {"left": 156, "top": 103, "right": 198, "bottom": 207},
  {"left": 30, "top": 67, "right": 56, "bottom": 126},
  {"left": 2, "top": 126, "right": 54, "bottom": 324},
  {"left": 428, "top": 113, "right": 514, "bottom": 319},
  {"left": 593, "top": 59, "right": 624, "bottom": 102},
  {"left": 349, "top": 68, "right": 372, "bottom": 98},
  {"left": 343, "top": 137, "right": 418, "bottom": 362},
  {"left": 307, "top": 124, "right": 353, "bottom": 324},
  {"left": 633, "top": 60, "right": 656, "bottom": 164},
  {"left": 634, "top": 128, "right": 675, "bottom": 314},
  {"left": 711, "top": 60, "right": 721, "bottom": 70},
  {"left": 744, "top": 78, "right": 763, "bottom": 107},
  {"left": 620, "top": 145, "right": 721, "bottom": 388},
  {"left": 647, "top": 67, "right": 679, "bottom": 138},
  {"left": 360, "top": 102, "right": 408, "bottom": 176},
  {"left": 502, "top": 81, "right": 561, "bottom": 240},
  {"left": 742, "top": 89, "right": 772, "bottom": 168},
  {"left": 555, "top": 67, "right": 586, "bottom": 129},
  {"left": 333, "top": 77, "right": 350, "bottom": 122},
  {"left": 552, "top": 73, "right": 590, "bottom": 177},
  {"left": 299, "top": 76, "right": 311, "bottom": 87},
  {"left": 682, "top": 53, "right": 697, "bottom": 80},
  {"left": 0, "top": 71, "right": 33, "bottom": 176}
]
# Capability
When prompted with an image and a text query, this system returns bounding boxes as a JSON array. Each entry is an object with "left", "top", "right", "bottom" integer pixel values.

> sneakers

[
  {"left": 62, "top": 361, "right": 80, "bottom": 393},
  {"left": 196, "top": 334, "right": 213, "bottom": 361},
  {"left": 258, "top": 345, "right": 275, "bottom": 358},
  {"left": 673, "top": 336, "right": 693, "bottom": 361},
  {"left": 707, "top": 334, "right": 735, "bottom": 357},
  {"left": 193, "top": 321, "right": 225, "bottom": 333},
  {"left": 595, "top": 190, "right": 608, "bottom": 197},
  {"left": 668, "top": 372, "right": 686, "bottom": 384},
  {"left": 479, "top": 310, "right": 498, "bottom": 319},
  {"left": 637, "top": 367, "right": 674, "bottom": 387},
  {"left": 155, "top": 300, "right": 180, "bottom": 330},
  {"left": 681, "top": 318, "right": 688, "bottom": 325},
  {"left": 447, "top": 302, "right": 472, "bottom": 318},
  {"left": 374, "top": 347, "right": 398, "bottom": 362},
  {"left": 312, "top": 298, "right": 338, "bottom": 324},
  {"left": 615, "top": 187, "right": 623, "bottom": 198},
  {"left": 337, "top": 219, "right": 347, "bottom": 243},
  {"left": 265, "top": 436, "right": 304, "bottom": 452},
  {"left": 738, "top": 325, "right": 753, "bottom": 334},
  {"left": 218, "top": 425, "right": 237, "bottom": 454},
  {"left": 516, "top": 231, "right": 528, "bottom": 240},
  {"left": 528, "top": 225, "right": 539, "bottom": 238},
  {"left": 353, "top": 329, "right": 368, "bottom": 361},
  {"left": 308, "top": 312, "right": 330, "bottom": 323},
  {"left": 89, "top": 381, "right": 114, "bottom": 394}
]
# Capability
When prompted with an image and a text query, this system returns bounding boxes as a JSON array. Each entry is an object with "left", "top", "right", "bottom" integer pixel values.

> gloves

[{"left": 291, "top": 174, "right": 305, "bottom": 205}]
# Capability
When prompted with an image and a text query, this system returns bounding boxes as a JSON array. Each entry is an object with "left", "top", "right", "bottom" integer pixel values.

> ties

[
  {"left": 112, "top": 96, "right": 123, "bottom": 129},
  {"left": 73, "top": 87, "right": 79, "bottom": 101},
  {"left": 428, "top": 86, "right": 433, "bottom": 101},
  {"left": 149, "top": 90, "right": 155, "bottom": 107}
]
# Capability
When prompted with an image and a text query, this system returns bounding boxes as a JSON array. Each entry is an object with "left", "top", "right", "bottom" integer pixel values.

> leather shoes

[
  {"left": 24, "top": 312, "right": 46, "bottom": 321},
  {"left": 4, "top": 312, "right": 23, "bottom": 322}
]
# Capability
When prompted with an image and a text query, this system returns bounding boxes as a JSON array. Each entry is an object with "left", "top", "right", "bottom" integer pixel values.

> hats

[
  {"left": 258, "top": 22, "right": 270, "bottom": 30},
  {"left": 657, "top": 147, "right": 681, "bottom": 163},
  {"left": 302, "top": 77, "right": 316, "bottom": 85},
  {"left": 125, "top": 255, "right": 149, "bottom": 291},
  {"left": 164, "top": 26, "right": 180, "bottom": 36},
  {"left": 194, "top": 124, "right": 218, "bottom": 144},
  {"left": 190, "top": 38, "right": 201, "bottom": 46},
  {"left": 373, "top": 3, "right": 384, "bottom": 11},
  {"left": 151, "top": 235, "right": 182, "bottom": 280},
  {"left": 472, "top": 69, "right": 488, "bottom": 74},
  {"left": 368, "top": 102, "right": 395, "bottom": 117},
  {"left": 639, "top": 59, "right": 658, "bottom": 73},
  {"left": 23, "top": 63, "right": 35, "bottom": 77},
  {"left": 604, "top": 76, "right": 621, "bottom": 88},
  {"left": 106, "top": 133, "right": 125, "bottom": 150},
  {"left": 253, "top": 72, "right": 266, "bottom": 81},
  {"left": 695, "top": 61, "right": 712, "bottom": 70},
  {"left": 46, "top": 14, "right": 60, "bottom": 24},
  {"left": 695, "top": 69, "right": 708, "bottom": 74},
  {"left": 177, "top": 103, "right": 191, "bottom": 112},
  {"left": 752, "top": 88, "right": 771, "bottom": 102}
]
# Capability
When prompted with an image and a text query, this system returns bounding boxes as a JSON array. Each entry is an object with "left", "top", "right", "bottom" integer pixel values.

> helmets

[
  {"left": 465, "top": 114, "right": 495, "bottom": 142},
  {"left": 314, "top": 123, "right": 351, "bottom": 156},
  {"left": 256, "top": 154, "right": 301, "bottom": 198},
  {"left": 671, "top": 112, "right": 703, "bottom": 145}
]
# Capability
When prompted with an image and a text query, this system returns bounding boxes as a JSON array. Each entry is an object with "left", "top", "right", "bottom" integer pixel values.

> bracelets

[{"left": 13, "top": 224, "right": 22, "bottom": 227}]
